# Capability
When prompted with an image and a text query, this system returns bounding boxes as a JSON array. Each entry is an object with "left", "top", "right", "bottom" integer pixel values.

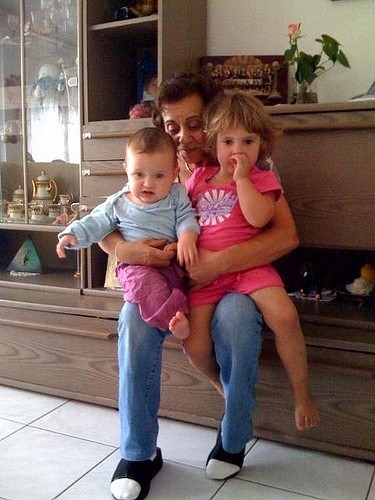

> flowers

[{"left": 283, "top": 21, "right": 351, "bottom": 85}]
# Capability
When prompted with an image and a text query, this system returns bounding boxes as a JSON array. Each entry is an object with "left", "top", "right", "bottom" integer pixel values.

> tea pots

[{"left": 30, "top": 170, "right": 58, "bottom": 215}]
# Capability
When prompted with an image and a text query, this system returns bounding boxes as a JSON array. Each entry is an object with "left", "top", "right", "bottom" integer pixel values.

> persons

[
  {"left": 99, "top": 67, "right": 301, "bottom": 500},
  {"left": 57, "top": 127, "right": 201, "bottom": 339},
  {"left": 182, "top": 91, "right": 321, "bottom": 432}
]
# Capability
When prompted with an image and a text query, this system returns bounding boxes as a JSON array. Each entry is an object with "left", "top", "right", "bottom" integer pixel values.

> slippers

[
  {"left": 109, "top": 448, "right": 165, "bottom": 500},
  {"left": 204, "top": 410, "right": 248, "bottom": 481}
]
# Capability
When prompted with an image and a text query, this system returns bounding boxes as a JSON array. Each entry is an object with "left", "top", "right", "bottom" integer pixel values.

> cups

[
  {"left": 9, "top": 204, "right": 24, "bottom": 221},
  {"left": 48, "top": 204, "right": 61, "bottom": 218},
  {"left": 59, "top": 193, "right": 74, "bottom": 206},
  {"left": 30, "top": 206, "right": 46, "bottom": 221}
]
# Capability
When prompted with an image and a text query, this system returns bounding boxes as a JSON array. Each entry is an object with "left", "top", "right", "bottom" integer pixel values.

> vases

[{"left": 295, "top": 80, "right": 308, "bottom": 103}]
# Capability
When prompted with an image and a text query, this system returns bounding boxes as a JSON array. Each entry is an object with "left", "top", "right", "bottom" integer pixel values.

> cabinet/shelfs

[{"left": 0, "top": 0, "right": 375, "bottom": 465}]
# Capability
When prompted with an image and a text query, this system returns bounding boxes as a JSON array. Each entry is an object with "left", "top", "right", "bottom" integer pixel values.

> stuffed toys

[
  {"left": 31, "top": 64, "right": 70, "bottom": 99},
  {"left": 129, "top": 73, "right": 159, "bottom": 119}
]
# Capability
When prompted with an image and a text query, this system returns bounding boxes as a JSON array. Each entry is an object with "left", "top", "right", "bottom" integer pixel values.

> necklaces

[{"left": 184, "top": 162, "right": 193, "bottom": 174}]
